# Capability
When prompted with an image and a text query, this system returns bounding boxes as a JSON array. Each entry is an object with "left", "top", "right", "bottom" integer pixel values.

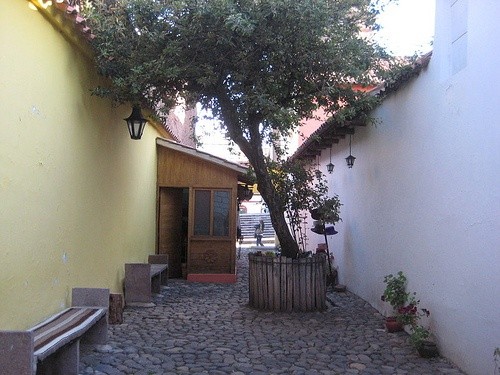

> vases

[
  {"left": 419, "top": 340, "right": 437, "bottom": 359},
  {"left": 384, "top": 314, "right": 402, "bottom": 333}
]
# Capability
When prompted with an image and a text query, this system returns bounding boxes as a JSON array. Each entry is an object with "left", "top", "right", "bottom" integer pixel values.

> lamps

[
  {"left": 315, "top": 155, "right": 322, "bottom": 178},
  {"left": 345, "top": 133, "right": 356, "bottom": 169},
  {"left": 326, "top": 145, "right": 335, "bottom": 175},
  {"left": 113, "top": 97, "right": 149, "bottom": 140}
]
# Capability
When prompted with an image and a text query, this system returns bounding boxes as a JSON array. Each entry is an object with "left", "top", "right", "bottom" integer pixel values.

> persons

[{"left": 255, "top": 220, "right": 264, "bottom": 246}]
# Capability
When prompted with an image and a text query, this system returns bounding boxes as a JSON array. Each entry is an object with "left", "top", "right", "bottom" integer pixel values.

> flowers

[{"left": 381, "top": 272, "right": 434, "bottom": 354}]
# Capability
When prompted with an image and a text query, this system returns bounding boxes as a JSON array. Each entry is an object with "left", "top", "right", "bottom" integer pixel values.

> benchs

[
  {"left": 0, "top": 288, "right": 111, "bottom": 375},
  {"left": 124, "top": 253, "right": 168, "bottom": 307}
]
